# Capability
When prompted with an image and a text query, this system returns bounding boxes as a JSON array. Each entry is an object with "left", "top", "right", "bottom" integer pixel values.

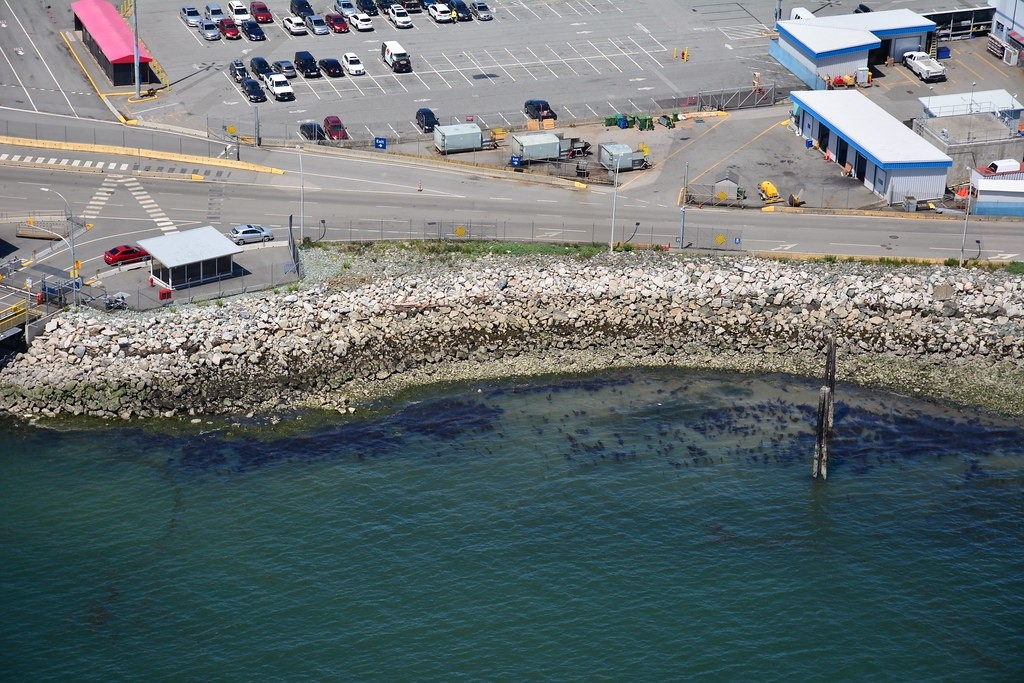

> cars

[
  {"left": 240, "top": 77, "right": 267, "bottom": 102},
  {"left": 319, "top": 58, "right": 344, "bottom": 77},
  {"left": 104, "top": 244, "right": 151, "bottom": 267},
  {"left": 300, "top": 122, "right": 325, "bottom": 140},
  {"left": 179, "top": 0, "right": 494, "bottom": 41},
  {"left": 229, "top": 224, "right": 274, "bottom": 246},
  {"left": 525, "top": 99, "right": 557, "bottom": 122},
  {"left": 272, "top": 60, "right": 297, "bottom": 78},
  {"left": 251, "top": 56, "right": 273, "bottom": 77},
  {"left": 342, "top": 52, "right": 365, "bottom": 76}
]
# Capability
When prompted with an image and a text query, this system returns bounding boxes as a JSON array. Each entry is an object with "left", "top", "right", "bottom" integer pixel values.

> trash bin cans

[
  {"left": 605, "top": 113, "right": 635, "bottom": 129},
  {"left": 672, "top": 111, "right": 678, "bottom": 122}
]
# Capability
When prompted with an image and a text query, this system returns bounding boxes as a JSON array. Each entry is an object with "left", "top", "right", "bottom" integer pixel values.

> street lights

[{"left": 27, "top": 188, "right": 76, "bottom": 307}]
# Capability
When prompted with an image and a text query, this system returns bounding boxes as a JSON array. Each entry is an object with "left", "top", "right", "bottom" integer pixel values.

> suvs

[
  {"left": 229, "top": 60, "right": 251, "bottom": 83},
  {"left": 324, "top": 116, "right": 347, "bottom": 140},
  {"left": 294, "top": 51, "right": 321, "bottom": 78},
  {"left": 416, "top": 108, "right": 440, "bottom": 133}
]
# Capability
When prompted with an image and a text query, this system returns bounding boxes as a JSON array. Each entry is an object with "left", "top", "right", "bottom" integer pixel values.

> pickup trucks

[
  {"left": 263, "top": 71, "right": 294, "bottom": 101},
  {"left": 902, "top": 50, "right": 947, "bottom": 82}
]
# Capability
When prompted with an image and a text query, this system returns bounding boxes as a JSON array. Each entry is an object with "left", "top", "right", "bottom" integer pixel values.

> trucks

[{"left": 381, "top": 41, "right": 411, "bottom": 73}]
